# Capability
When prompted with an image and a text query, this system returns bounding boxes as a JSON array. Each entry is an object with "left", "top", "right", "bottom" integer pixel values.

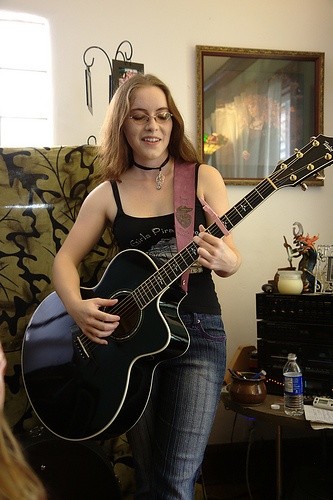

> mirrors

[{"left": 195, "top": 45, "right": 324, "bottom": 187}]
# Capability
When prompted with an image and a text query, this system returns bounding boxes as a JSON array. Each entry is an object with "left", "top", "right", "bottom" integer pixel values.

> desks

[{"left": 221, "top": 391, "right": 312, "bottom": 500}]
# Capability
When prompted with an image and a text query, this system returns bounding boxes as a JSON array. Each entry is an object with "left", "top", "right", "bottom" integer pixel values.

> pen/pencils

[{"left": 228, "top": 367, "right": 247, "bottom": 380}]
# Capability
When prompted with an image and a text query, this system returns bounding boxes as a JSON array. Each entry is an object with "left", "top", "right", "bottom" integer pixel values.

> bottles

[{"left": 283, "top": 354, "right": 304, "bottom": 416}]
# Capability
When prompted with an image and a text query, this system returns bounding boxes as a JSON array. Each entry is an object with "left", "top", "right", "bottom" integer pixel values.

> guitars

[{"left": 21, "top": 132, "right": 333, "bottom": 442}]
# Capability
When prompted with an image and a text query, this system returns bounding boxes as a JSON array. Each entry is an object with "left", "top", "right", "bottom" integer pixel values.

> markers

[{"left": 251, "top": 370, "right": 267, "bottom": 379}]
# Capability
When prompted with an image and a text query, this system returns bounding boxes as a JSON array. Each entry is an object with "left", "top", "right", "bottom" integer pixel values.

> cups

[{"left": 278, "top": 270, "right": 303, "bottom": 294}]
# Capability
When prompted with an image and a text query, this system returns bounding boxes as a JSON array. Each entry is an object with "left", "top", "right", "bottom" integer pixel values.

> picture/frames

[{"left": 112, "top": 59, "right": 144, "bottom": 98}]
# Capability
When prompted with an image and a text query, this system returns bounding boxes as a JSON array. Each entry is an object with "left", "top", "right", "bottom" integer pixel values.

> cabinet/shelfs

[{"left": 256, "top": 293, "right": 333, "bottom": 396}]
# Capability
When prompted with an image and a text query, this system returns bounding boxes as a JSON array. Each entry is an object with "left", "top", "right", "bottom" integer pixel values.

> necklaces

[{"left": 131, "top": 152, "right": 170, "bottom": 190}]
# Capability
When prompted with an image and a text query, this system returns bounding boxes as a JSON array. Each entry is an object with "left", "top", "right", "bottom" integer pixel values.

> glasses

[{"left": 127, "top": 111, "right": 174, "bottom": 124}]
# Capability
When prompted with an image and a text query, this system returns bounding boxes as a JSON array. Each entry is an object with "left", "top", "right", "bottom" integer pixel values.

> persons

[{"left": 52, "top": 74, "right": 241, "bottom": 500}]
